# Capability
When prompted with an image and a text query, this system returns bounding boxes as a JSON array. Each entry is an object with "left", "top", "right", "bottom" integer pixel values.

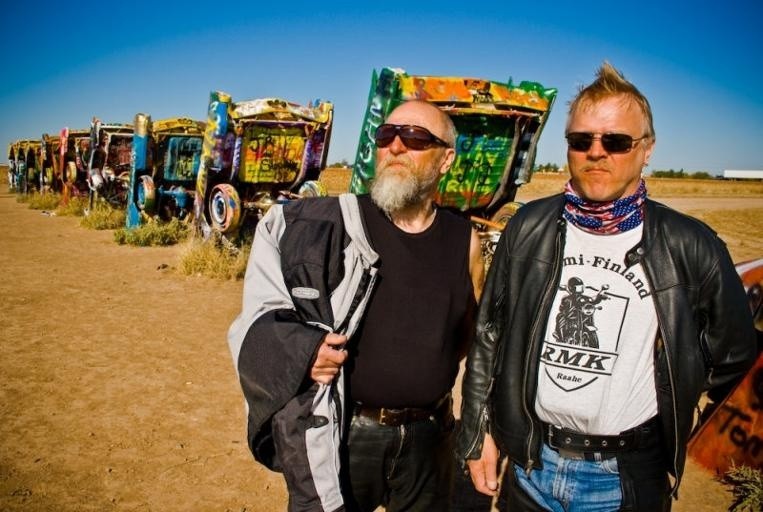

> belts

[
  {"left": 542, "top": 428, "right": 654, "bottom": 460},
  {"left": 356, "top": 403, "right": 448, "bottom": 425}
]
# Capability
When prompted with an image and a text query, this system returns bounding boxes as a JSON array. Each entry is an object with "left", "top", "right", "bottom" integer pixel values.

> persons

[
  {"left": 458, "top": 62, "right": 759, "bottom": 512},
  {"left": 225, "top": 100, "right": 493, "bottom": 512}
]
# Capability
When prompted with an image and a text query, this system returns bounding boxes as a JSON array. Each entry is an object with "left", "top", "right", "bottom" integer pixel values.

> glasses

[
  {"left": 374, "top": 123, "right": 450, "bottom": 151},
  {"left": 564, "top": 132, "right": 646, "bottom": 153}
]
